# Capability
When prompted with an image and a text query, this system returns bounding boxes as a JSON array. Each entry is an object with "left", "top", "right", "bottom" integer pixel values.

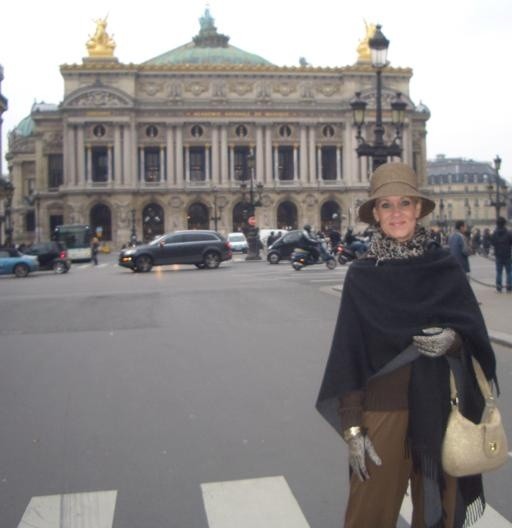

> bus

[{"left": 53, "top": 222, "right": 94, "bottom": 262}]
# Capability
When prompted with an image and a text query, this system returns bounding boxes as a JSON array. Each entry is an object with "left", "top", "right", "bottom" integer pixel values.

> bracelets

[{"left": 341, "top": 422, "right": 370, "bottom": 444}]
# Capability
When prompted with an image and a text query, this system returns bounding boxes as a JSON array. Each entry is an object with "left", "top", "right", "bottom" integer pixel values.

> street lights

[
  {"left": 350, "top": 20, "right": 408, "bottom": 179},
  {"left": 3, "top": 181, "right": 18, "bottom": 247},
  {"left": 484, "top": 156, "right": 512, "bottom": 226},
  {"left": 130, "top": 207, "right": 137, "bottom": 236},
  {"left": 208, "top": 189, "right": 224, "bottom": 230},
  {"left": 238, "top": 153, "right": 270, "bottom": 265}
]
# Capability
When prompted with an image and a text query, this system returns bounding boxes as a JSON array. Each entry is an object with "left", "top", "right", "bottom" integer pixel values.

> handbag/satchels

[{"left": 442, "top": 404, "right": 509, "bottom": 478}]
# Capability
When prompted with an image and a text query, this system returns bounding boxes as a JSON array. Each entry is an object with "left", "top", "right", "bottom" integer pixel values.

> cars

[
  {"left": 266, "top": 229, "right": 308, "bottom": 264},
  {"left": 227, "top": 232, "right": 249, "bottom": 255},
  {"left": 0, "top": 246, "right": 41, "bottom": 277},
  {"left": 18, "top": 241, "right": 70, "bottom": 274}
]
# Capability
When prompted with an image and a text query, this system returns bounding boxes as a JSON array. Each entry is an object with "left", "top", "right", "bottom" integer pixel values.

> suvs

[{"left": 118, "top": 229, "right": 231, "bottom": 273}]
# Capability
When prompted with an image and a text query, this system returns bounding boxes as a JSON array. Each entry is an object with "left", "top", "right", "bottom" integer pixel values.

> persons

[{"left": 316, "top": 162, "right": 498, "bottom": 527}]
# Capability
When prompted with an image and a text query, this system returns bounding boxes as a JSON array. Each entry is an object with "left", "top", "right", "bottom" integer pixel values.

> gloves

[
  {"left": 346, "top": 431, "right": 382, "bottom": 483},
  {"left": 412, "top": 327, "right": 457, "bottom": 359}
]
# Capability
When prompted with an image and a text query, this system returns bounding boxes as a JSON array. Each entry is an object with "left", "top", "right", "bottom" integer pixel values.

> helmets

[
  {"left": 348, "top": 227, "right": 352, "bottom": 232},
  {"left": 303, "top": 224, "right": 310, "bottom": 231}
]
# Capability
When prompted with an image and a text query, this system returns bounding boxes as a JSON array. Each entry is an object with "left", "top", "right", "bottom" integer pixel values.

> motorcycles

[
  {"left": 337, "top": 239, "right": 371, "bottom": 264},
  {"left": 291, "top": 234, "right": 336, "bottom": 270}
]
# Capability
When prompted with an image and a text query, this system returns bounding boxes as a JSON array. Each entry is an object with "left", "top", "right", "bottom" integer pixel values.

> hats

[{"left": 359, "top": 162, "right": 436, "bottom": 225}]
